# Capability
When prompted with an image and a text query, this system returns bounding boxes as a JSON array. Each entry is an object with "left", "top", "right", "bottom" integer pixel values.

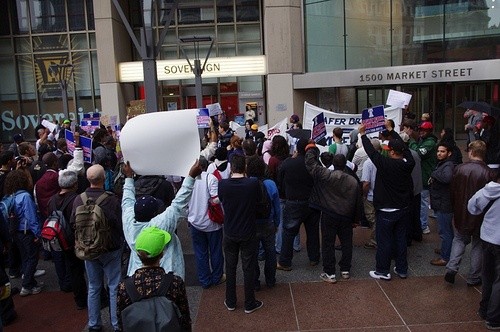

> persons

[
  {"left": 246, "top": 104, "right": 255, "bottom": 121},
  {"left": 428, "top": 109, "right": 500, "bottom": 329},
  {"left": 218, "top": 154, "right": 271, "bottom": 313},
  {"left": 70, "top": 165, "right": 122, "bottom": 332},
  {"left": 117, "top": 227, "right": 192, "bottom": 332},
  {"left": 0, "top": 111, "right": 438, "bottom": 332}
]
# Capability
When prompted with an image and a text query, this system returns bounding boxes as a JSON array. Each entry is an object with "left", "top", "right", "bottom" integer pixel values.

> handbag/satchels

[{"left": 208, "top": 195, "right": 224, "bottom": 224}]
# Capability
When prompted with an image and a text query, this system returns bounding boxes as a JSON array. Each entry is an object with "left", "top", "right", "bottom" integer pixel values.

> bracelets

[{"left": 75, "top": 146, "right": 80, "bottom": 147}]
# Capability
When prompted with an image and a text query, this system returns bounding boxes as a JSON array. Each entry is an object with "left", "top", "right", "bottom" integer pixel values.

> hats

[
  {"left": 42, "top": 151, "right": 62, "bottom": 166},
  {"left": 134, "top": 227, "right": 171, "bottom": 258},
  {"left": 422, "top": 122, "right": 433, "bottom": 132},
  {"left": 384, "top": 139, "right": 404, "bottom": 151},
  {"left": 13, "top": 133, "right": 24, "bottom": 141},
  {"left": 135, "top": 195, "right": 163, "bottom": 220},
  {"left": 64, "top": 119, "right": 70, "bottom": 124}
]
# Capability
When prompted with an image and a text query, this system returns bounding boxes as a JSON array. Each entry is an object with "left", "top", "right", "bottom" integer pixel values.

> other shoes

[
  {"left": 444, "top": 273, "right": 455, "bottom": 283},
  {"left": 435, "top": 248, "right": 441, "bottom": 253},
  {"left": 319, "top": 273, "right": 336, "bottom": 283},
  {"left": 468, "top": 277, "right": 483, "bottom": 287},
  {"left": 10, "top": 270, "right": 45, "bottom": 280},
  {"left": 369, "top": 271, "right": 393, "bottom": 280},
  {"left": 341, "top": 272, "right": 349, "bottom": 279},
  {"left": 431, "top": 257, "right": 448, "bottom": 265},
  {"left": 334, "top": 245, "right": 342, "bottom": 250},
  {"left": 19, "top": 282, "right": 44, "bottom": 296},
  {"left": 102, "top": 300, "right": 110, "bottom": 309},
  {"left": 202, "top": 247, "right": 320, "bottom": 313},
  {"left": 423, "top": 226, "right": 430, "bottom": 234},
  {"left": 363, "top": 243, "right": 377, "bottom": 249},
  {"left": 393, "top": 266, "right": 407, "bottom": 279}
]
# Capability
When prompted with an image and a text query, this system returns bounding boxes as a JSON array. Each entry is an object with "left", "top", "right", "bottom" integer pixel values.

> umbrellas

[{"left": 463, "top": 101, "right": 496, "bottom": 116}]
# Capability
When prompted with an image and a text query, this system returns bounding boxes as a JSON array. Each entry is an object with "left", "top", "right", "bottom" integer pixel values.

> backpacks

[
  {"left": 74, "top": 193, "right": 108, "bottom": 260},
  {"left": 41, "top": 193, "right": 72, "bottom": 252},
  {"left": 120, "top": 274, "right": 177, "bottom": 332}
]
